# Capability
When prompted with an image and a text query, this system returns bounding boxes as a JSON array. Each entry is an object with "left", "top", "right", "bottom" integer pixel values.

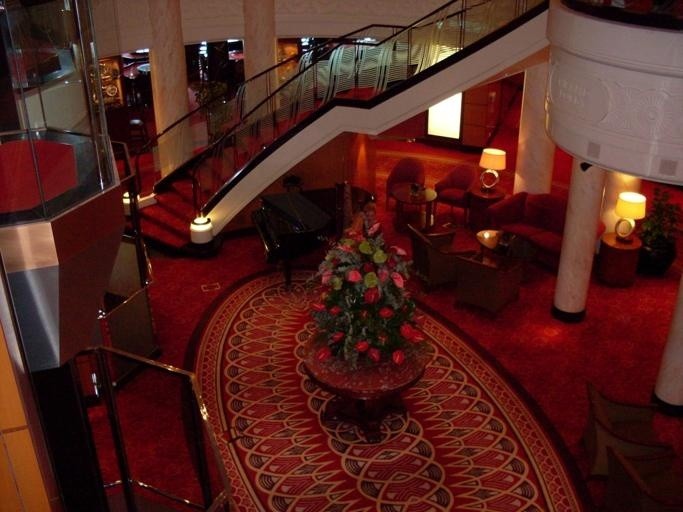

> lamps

[
  {"left": 616, "top": 190, "right": 647, "bottom": 244},
  {"left": 189, "top": 216, "right": 214, "bottom": 246},
  {"left": 122, "top": 191, "right": 139, "bottom": 216},
  {"left": 476, "top": 148, "right": 508, "bottom": 193}
]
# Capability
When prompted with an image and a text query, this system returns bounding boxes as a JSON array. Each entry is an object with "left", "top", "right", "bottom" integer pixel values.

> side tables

[{"left": 598, "top": 232, "right": 643, "bottom": 289}]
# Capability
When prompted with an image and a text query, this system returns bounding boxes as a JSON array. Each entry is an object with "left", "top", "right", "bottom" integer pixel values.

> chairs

[
  {"left": 378, "top": 155, "right": 525, "bottom": 318},
  {"left": 574, "top": 381, "right": 683, "bottom": 512}
]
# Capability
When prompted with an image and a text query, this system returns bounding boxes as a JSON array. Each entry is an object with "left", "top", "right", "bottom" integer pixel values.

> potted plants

[{"left": 639, "top": 186, "right": 682, "bottom": 277}]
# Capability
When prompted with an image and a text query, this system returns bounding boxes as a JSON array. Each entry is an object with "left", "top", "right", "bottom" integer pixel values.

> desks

[{"left": 301, "top": 331, "right": 426, "bottom": 428}]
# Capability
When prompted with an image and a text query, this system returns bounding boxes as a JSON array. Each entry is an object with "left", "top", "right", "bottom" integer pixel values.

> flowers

[{"left": 301, "top": 221, "right": 429, "bottom": 370}]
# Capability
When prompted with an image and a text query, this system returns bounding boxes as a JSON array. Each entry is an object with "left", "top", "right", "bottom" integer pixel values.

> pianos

[{"left": 250, "top": 183, "right": 376, "bottom": 292}]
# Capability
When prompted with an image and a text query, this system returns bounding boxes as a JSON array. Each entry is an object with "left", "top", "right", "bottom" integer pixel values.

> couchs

[{"left": 476, "top": 191, "right": 605, "bottom": 277}]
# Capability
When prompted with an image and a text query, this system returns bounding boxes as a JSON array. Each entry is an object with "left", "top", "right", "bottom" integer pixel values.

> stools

[{"left": 130, "top": 116, "right": 149, "bottom": 157}]
